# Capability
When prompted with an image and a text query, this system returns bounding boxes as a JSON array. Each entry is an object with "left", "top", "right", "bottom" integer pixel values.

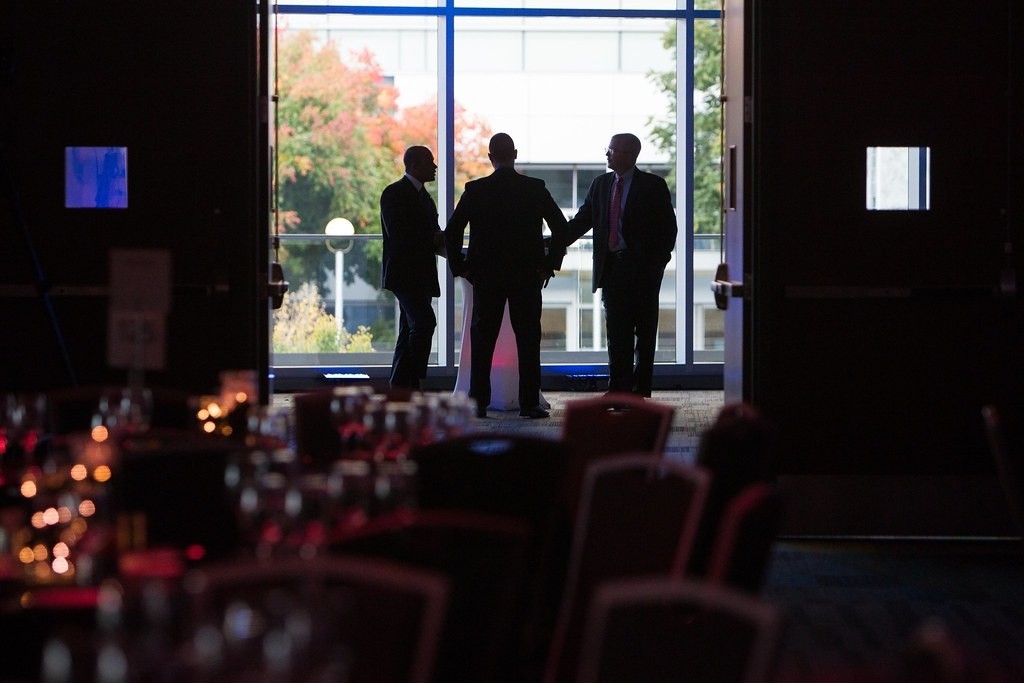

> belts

[{"left": 608, "top": 250, "right": 627, "bottom": 259}]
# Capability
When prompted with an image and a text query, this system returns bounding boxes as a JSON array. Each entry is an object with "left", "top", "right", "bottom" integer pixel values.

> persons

[
  {"left": 444, "top": 133, "right": 570, "bottom": 420},
  {"left": 378, "top": 146, "right": 449, "bottom": 401},
  {"left": 544, "top": 134, "right": 677, "bottom": 409}
]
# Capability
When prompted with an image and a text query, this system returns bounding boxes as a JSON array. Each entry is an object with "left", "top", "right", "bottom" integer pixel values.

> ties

[
  {"left": 608, "top": 177, "right": 623, "bottom": 249},
  {"left": 421, "top": 186, "right": 430, "bottom": 200}
]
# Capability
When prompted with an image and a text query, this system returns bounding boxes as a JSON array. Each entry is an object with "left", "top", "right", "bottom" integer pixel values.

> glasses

[{"left": 604, "top": 147, "right": 636, "bottom": 156}]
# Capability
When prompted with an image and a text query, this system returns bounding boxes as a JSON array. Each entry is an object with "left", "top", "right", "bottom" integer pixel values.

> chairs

[{"left": 176, "top": 382, "right": 787, "bottom": 682}]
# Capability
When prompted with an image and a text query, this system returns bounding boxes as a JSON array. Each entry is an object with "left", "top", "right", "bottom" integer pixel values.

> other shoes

[
  {"left": 471, "top": 408, "right": 486, "bottom": 417},
  {"left": 519, "top": 406, "right": 549, "bottom": 418}
]
